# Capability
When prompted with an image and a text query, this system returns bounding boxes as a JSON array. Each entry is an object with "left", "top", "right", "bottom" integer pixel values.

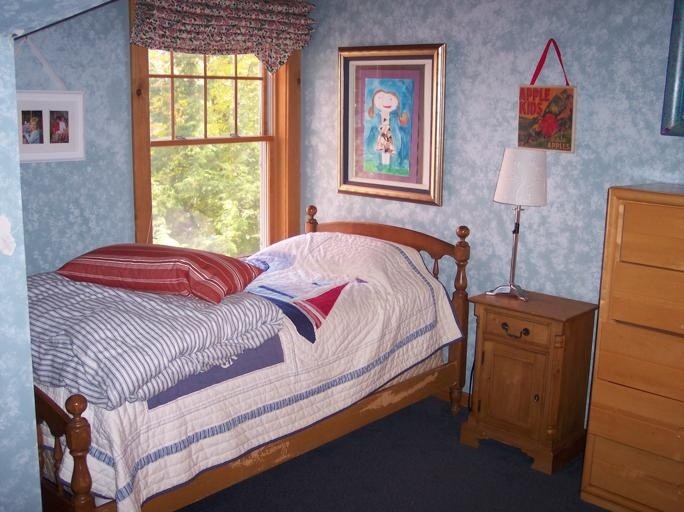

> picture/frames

[
  {"left": 337, "top": 43, "right": 446, "bottom": 207},
  {"left": 516, "top": 84, "right": 579, "bottom": 154},
  {"left": 16, "top": 89, "right": 87, "bottom": 164},
  {"left": 661, "top": 0, "right": 684, "bottom": 137}
]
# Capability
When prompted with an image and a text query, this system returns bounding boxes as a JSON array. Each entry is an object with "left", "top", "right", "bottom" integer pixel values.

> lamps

[{"left": 485, "top": 147, "right": 547, "bottom": 301}]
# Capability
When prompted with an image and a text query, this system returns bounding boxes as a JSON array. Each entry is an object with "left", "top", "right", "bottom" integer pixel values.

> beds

[{"left": 26, "top": 206, "right": 470, "bottom": 512}]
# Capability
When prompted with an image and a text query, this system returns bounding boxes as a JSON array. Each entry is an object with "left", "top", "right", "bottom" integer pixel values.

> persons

[
  {"left": 50, "top": 114, "right": 70, "bottom": 143},
  {"left": 22, "top": 116, "right": 42, "bottom": 144}
]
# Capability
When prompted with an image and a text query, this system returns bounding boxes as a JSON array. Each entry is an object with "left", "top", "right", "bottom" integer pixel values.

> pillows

[{"left": 55, "top": 244, "right": 264, "bottom": 305}]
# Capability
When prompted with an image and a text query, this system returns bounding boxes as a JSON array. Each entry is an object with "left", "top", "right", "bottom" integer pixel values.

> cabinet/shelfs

[{"left": 579, "top": 181, "right": 684, "bottom": 511}]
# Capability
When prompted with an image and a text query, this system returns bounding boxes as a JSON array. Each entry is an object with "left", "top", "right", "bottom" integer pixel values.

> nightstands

[{"left": 459, "top": 291, "right": 598, "bottom": 476}]
